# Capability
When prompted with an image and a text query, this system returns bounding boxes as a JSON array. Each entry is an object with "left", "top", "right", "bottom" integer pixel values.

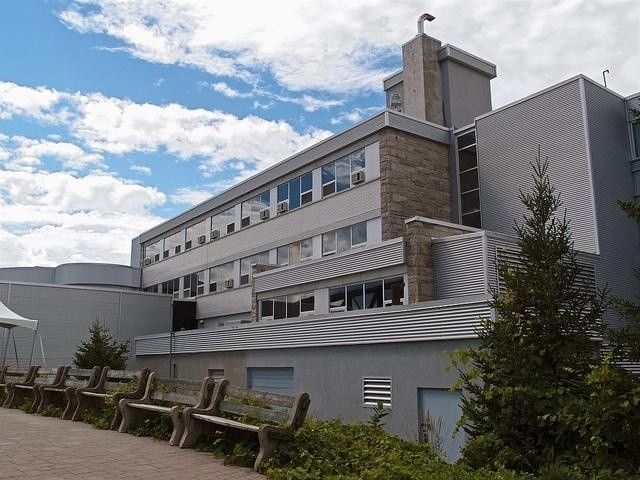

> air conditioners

[
  {"left": 224, "top": 279, "right": 232, "bottom": 288},
  {"left": 259, "top": 202, "right": 289, "bottom": 220},
  {"left": 351, "top": 170, "right": 365, "bottom": 185},
  {"left": 144, "top": 258, "right": 151, "bottom": 264},
  {"left": 198, "top": 229, "right": 220, "bottom": 244}
]
